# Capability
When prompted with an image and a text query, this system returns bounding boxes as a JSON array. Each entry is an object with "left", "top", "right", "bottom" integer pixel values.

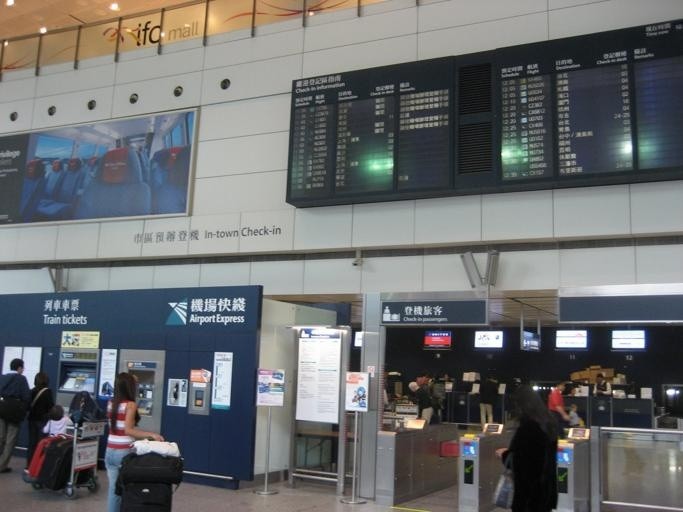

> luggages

[{"left": 120, "top": 484, "right": 173, "bottom": 511}]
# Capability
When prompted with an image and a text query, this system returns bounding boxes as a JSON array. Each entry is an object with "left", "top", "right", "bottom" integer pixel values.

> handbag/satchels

[
  {"left": 117, "top": 439, "right": 184, "bottom": 485},
  {"left": 492, "top": 451, "right": 514, "bottom": 509},
  {"left": 27, "top": 434, "right": 75, "bottom": 490},
  {"left": 0, "top": 374, "right": 27, "bottom": 423}
]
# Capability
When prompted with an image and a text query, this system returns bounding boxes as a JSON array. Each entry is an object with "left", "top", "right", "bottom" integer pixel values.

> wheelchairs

[{"left": 22, "top": 415, "right": 105, "bottom": 499}]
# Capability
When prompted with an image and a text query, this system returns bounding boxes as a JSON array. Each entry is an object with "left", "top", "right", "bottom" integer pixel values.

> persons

[
  {"left": 0, "top": 358, "right": 31, "bottom": 474},
  {"left": 39, "top": 404, "right": 75, "bottom": 435},
  {"left": 23, "top": 371, "right": 54, "bottom": 474},
  {"left": 493, "top": 381, "right": 565, "bottom": 510},
  {"left": 383, "top": 369, "right": 612, "bottom": 427},
  {"left": 104, "top": 372, "right": 165, "bottom": 512}
]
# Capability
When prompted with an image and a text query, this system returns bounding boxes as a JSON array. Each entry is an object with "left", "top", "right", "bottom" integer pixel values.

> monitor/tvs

[
  {"left": 608, "top": 327, "right": 648, "bottom": 352},
  {"left": 136, "top": 399, "right": 152, "bottom": 414},
  {"left": 421, "top": 329, "right": 454, "bottom": 351},
  {"left": 552, "top": 327, "right": 591, "bottom": 352},
  {"left": 61, "top": 371, "right": 96, "bottom": 392},
  {"left": 472, "top": 328, "right": 506, "bottom": 352}
]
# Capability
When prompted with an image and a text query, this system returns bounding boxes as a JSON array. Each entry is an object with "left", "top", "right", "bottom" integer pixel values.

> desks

[{"left": 295, "top": 430, "right": 359, "bottom": 487}]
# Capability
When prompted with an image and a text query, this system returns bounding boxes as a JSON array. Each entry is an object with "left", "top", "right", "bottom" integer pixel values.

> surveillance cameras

[{"left": 352, "top": 259, "right": 362, "bottom": 266}]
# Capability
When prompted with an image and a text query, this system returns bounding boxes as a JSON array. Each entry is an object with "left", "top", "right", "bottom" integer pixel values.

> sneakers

[{"left": 1, "top": 466, "right": 12, "bottom": 472}]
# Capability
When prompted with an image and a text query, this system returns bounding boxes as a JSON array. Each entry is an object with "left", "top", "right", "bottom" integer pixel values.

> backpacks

[{"left": 69, "top": 391, "right": 101, "bottom": 424}]
